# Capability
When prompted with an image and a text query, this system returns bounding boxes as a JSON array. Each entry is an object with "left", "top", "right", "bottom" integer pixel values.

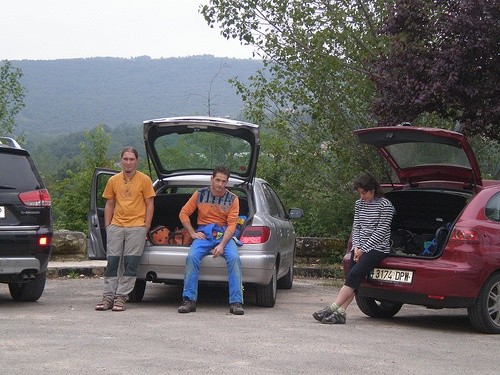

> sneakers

[
  {"left": 312, "top": 305, "right": 331, "bottom": 321},
  {"left": 178, "top": 299, "right": 197, "bottom": 313},
  {"left": 229, "top": 303, "right": 244, "bottom": 315},
  {"left": 321, "top": 311, "right": 347, "bottom": 324}
]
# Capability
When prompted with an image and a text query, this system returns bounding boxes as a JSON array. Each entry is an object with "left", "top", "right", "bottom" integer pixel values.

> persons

[
  {"left": 311, "top": 174, "right": 395, "bottom": 324},
  {"left": 178, "top": 166, "right": 244, "bottom": 315},
  {"left": 95, "top": 146, "right": 157, "bottom": 311}
]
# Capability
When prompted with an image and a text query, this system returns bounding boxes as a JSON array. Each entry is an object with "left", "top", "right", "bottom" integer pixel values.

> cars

[
  {"left": 87, "top": 116, "right": 306, "bottom": 308},
  {"left": 343, "top": 121, "right": 500, "bottom": 335}
]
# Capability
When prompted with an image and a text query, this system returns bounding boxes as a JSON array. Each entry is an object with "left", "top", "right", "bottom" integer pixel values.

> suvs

[{"left": 0, "top": 136, "right": 54, "bottom": 303}]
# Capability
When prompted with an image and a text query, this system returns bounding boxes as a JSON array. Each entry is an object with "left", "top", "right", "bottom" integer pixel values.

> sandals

[
  {"left": 95, "top": 300, "right": 112, "bottom": 310},
  {"left": 112, "top": 303, "right": 126, "bottom": 311}
]
